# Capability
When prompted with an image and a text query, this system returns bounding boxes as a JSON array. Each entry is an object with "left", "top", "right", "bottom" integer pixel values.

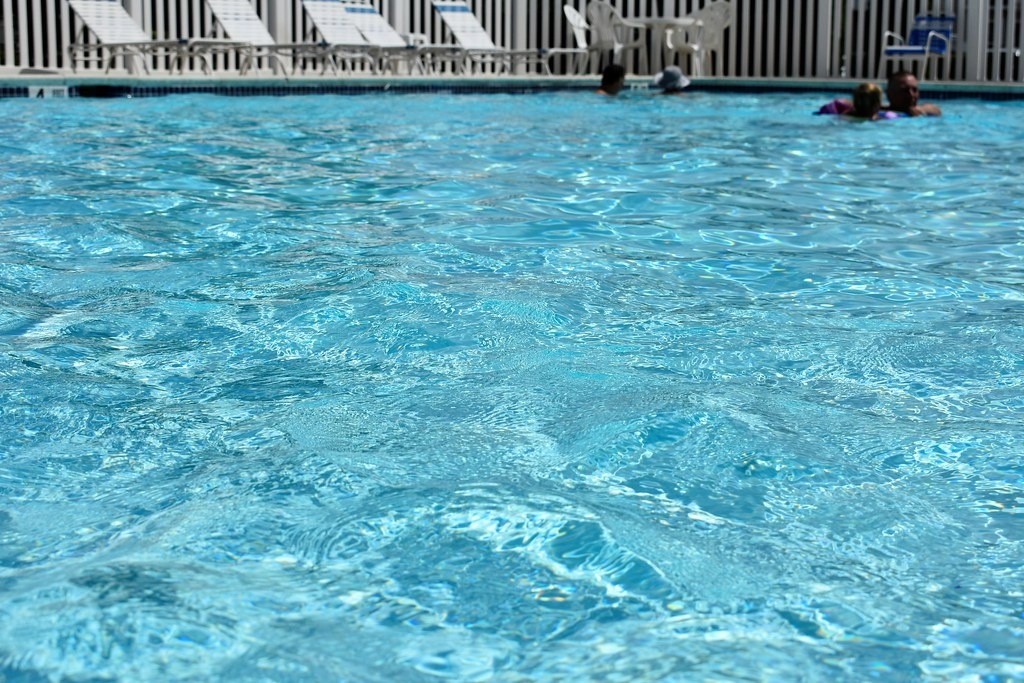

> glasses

[{"left": 889, "top": 85, "right": 917, "bottom": 93}]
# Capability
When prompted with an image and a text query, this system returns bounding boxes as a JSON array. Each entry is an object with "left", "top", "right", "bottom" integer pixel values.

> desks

[{"left": 624, "top": 16, "right": 702, "bottom": 74}]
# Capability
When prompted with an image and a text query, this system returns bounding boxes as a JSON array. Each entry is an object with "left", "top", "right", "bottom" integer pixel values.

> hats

[{"left": 658, "top": 65, "right": 691, "bottom": 88}]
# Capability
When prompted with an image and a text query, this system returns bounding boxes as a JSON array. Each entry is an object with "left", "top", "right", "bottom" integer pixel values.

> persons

[
  {"left": 880, "top": 71, "right": 942, "bottom": 115},
  {"left": 649, "top": 66, "right": 687, "bottom": 97},
  {"left": 595, "top": 65, "right": 627, "bottom": 96},
  {"left": 841, "top": 82, "right": 885, "bottom": 121}
]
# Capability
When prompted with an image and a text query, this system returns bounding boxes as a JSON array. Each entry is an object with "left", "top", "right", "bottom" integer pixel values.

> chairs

[
  {"left": 343, "top": 2, "right": 460, "bottom": 75},
  {"left": 65, "top": 0, "right": 193, "bottom": 80},
  {"left": 430, "top": 0, "right": 586, "bottom": 78},
  {"left": 197, "top": 0, "right": 321, "bottom": 82},
  {"left": 561, "top": 2, "right": 595, "bottom": 74},
  {"left": 299, "top": 0, "right": 426, "bottom": 78},
  {"left": 882, "top": 14, "right": 958, "bottom": 85},
  {"left": 587, "top": 0, "right": 649, "bottom": 74},
  {"left": 668, "top": 1, "right": 735, "bottom": 78}
]
